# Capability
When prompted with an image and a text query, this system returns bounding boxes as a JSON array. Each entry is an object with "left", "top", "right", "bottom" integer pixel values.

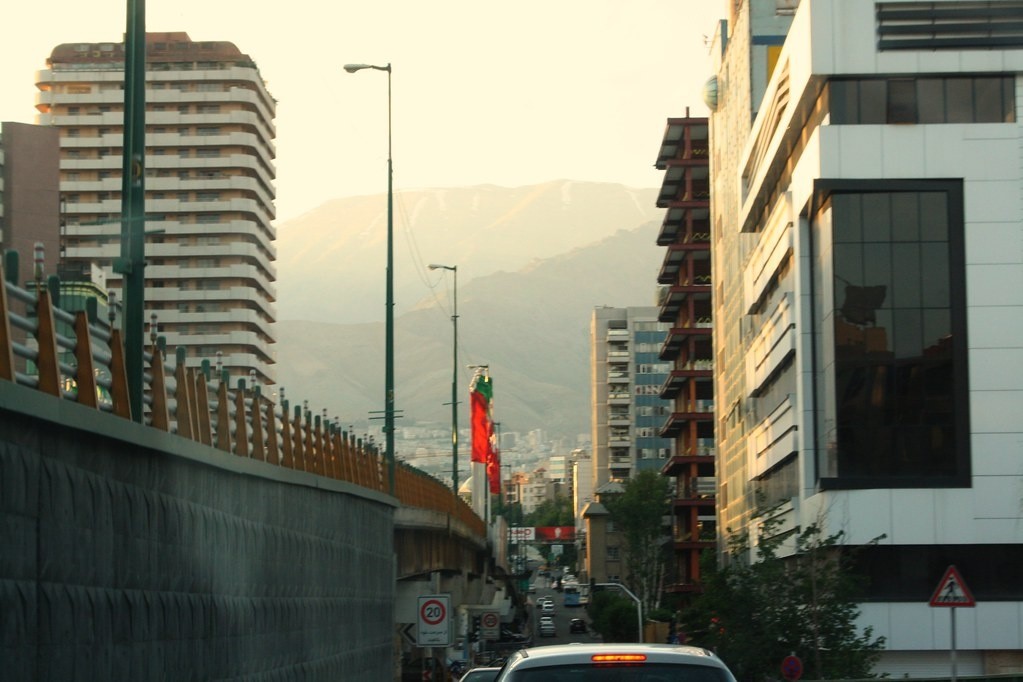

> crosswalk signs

[{"left": 926, "top": 564, "right": 976, "bottom": 608}]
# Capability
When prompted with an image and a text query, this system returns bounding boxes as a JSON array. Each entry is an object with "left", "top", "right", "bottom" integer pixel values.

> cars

[
  {"left": 569, "top": 618, "right": 586, "bottom": 633},
  {"left": 537, "top": 563, "right": 580, "bottom": 638},
  {"left": 527, "top": 583, "right": 537, "bottom": 594}
]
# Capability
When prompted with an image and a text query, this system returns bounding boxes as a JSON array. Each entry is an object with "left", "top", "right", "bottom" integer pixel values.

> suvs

[
  {"left": 493, "top": 641, "right": 737, "bottom": 682},
  {"left": 458, "top": 665, "right": 504, "bottom": 682}
]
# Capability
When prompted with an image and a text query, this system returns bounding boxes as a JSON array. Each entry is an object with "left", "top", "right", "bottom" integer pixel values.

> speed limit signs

[{"left": 419, "top": 599, "right": 445, "bottom": 624}]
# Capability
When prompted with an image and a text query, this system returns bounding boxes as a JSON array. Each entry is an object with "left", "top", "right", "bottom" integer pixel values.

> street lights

[
  {"left": 344, "top": 62, "right": 395, "bottom": 495},
  {"left": 428, "top": 264, "right": 459, "bottom": 493}
]
